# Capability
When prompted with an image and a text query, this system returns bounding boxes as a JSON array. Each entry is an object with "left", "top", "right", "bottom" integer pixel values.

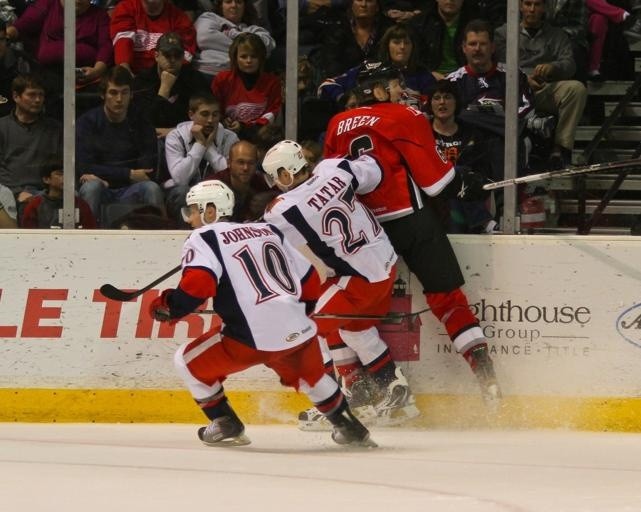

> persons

[
  {"left": 321, "top": 58, "right": 503, "bottom": 410},
  {"left": 1, "top": 0, "right": 633, "bottom": 231},
  {"left": 261, "top": 140, "right": 416, "bottom": 421},
  {"left": 148, "top": 178, "right": 371, "bottom": 445}
]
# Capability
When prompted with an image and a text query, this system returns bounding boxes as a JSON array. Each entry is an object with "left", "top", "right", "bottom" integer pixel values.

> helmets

[
  {"left": 185, "top": 178, "right": 234, "bottom": 226},
  {"left": 262, "top": 139, "right": 308, "bottom": 187},
  {"left": 353, "top": 58, "right": 400, "bottom": 104}
]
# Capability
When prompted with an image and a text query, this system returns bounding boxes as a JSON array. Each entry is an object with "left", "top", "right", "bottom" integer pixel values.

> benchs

[{"left": 529, "top": 36, "right": 641, "bottom": 235}]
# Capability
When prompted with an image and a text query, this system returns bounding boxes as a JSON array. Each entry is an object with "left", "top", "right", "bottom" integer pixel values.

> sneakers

[
  {"left": 620, "top": 12, "right": 638, "bottom": 30},
  {"left": 198, "top": 411, "right": 247, "bottom": 444},
  {"left": 590, "top": 72, "right": 605, "bottom": 83},
  {"left": 471, "top": 345, "right": 506, "bottom": 403},
  {"left": 484, "top": 220, "right": 503, "bottom": 233},
  {"left": 550, "top": 151, "right": 565, "bottom": 170},
  {"left": 297, "top": 367, "right": 415, "bottom": 445},
  {"left": 534, "top": 115, "right": 556, "bottom": 137}
]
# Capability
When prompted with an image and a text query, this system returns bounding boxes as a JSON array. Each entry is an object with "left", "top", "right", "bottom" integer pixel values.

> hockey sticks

[
  {"left": 189, "top": 308, "right": 432, "bottom": 320},
  {"left": 99, "top": 216, "right": 266, "bottom": 300}
]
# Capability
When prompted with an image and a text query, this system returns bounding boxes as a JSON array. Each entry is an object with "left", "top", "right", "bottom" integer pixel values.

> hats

[{"left": 156, "top": 32, "right": 183, "bottom": 54}]
